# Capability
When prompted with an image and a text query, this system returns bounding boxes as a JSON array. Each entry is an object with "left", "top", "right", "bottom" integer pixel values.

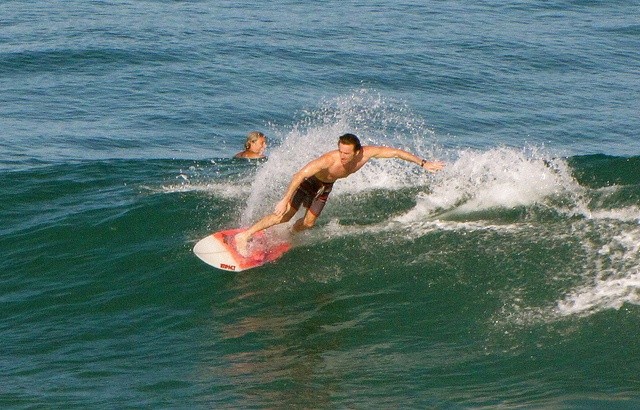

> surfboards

[{"left": 192, "top": 224, "right": 298, "bottom": 272}]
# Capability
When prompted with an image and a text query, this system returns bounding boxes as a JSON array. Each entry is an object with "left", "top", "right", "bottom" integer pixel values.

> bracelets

[{"left": 421, "top": 159, "right": 426, "bottom": 167}]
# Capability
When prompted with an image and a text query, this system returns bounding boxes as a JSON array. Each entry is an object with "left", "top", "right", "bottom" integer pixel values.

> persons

[
  {"left": 232, "top": 132, "right": 268, "bottom": 159},
  {"left": 235, "top": 134, "right": 445, "bottom": 260}
]
